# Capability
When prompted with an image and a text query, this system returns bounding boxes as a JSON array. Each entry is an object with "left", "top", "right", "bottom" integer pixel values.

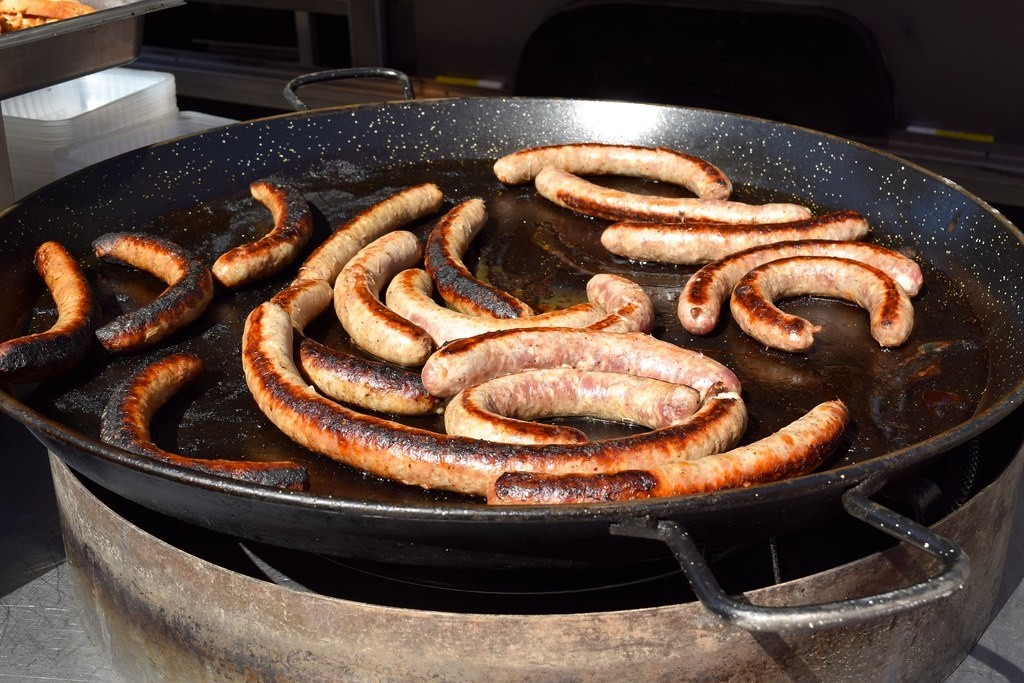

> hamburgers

[{"left": 0, "top": 0, "right": 95, "bottom": 36}]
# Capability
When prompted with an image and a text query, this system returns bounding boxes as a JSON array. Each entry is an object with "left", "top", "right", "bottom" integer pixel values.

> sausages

[{"left": 0, "top": 142, "right": 924, "bottom": 507}]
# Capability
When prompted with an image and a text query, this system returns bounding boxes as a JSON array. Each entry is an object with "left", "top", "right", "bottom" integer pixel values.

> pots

[{"left": 0, "top": 67, "right": 1024, "bottom": 632}]
